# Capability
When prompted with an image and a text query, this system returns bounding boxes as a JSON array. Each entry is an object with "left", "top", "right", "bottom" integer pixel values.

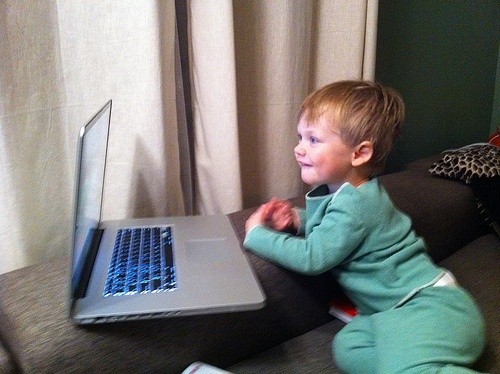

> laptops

[{"left": 68, "top": 101, "right": 264, "bottom": 326}]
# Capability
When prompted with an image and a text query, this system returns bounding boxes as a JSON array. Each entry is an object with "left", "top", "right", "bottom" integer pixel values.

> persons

[{"left": 241, "top": 78, "right": 488, "bottom": 374}]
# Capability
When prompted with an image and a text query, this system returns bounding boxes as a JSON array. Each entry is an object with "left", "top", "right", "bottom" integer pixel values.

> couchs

[{"left": 0, "top": 150, "right": 500, "bottom": 374}]
